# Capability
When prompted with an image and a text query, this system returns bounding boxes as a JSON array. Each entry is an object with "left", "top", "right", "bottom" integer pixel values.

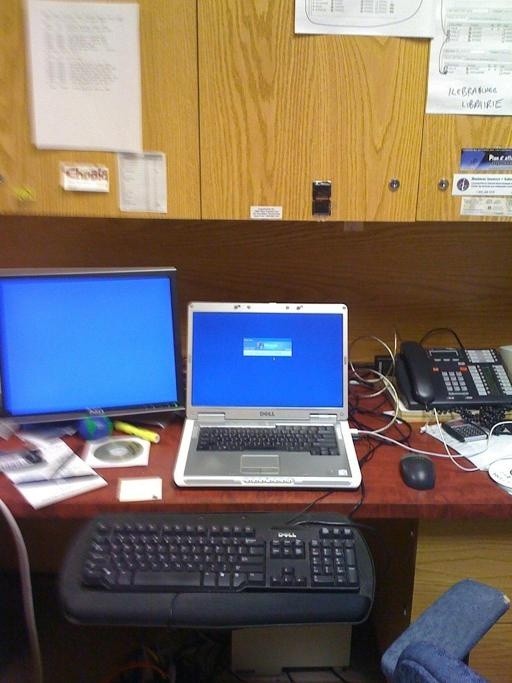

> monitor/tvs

[{"left": 0, "top": 265, "right": 186, "bottom": 426}]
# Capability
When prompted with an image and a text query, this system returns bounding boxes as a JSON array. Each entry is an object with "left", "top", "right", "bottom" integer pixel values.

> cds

[
  {"left": 487, "top": 457, "right": 512, "bottom": 488},
  {"left": 93, "top": 440, "right": 144, "bottom": 462}
]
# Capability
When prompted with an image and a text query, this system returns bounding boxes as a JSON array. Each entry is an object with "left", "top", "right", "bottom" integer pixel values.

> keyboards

[{"left": 60, "top": 510, "right": 375, "bottom": 627}]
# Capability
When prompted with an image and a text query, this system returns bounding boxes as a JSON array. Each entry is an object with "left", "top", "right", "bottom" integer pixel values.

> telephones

[{"left": 395, "top": 340, "right": 512, "bottom": 408}]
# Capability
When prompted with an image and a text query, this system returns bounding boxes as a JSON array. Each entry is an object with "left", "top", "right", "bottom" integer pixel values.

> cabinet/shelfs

[
  {"left": 196, "top": 0, "right": 512, "bottom": 225},
  {"left": 0, "top": 0, "right": 198, "bottom": 221}
]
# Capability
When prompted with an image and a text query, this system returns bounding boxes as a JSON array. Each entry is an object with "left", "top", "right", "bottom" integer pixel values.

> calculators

[{"left": 443, "top": 419, "right": 489, "bottom": 442}]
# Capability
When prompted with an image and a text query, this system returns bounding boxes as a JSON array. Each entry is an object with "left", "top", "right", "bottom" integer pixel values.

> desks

[{"left": 0, "top": 379, "right": 510, "bottom": 682}]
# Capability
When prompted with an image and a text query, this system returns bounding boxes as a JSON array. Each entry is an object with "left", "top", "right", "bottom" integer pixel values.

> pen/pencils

[{"left": 107, "top": 422, "right": 161, "bottom": 446}]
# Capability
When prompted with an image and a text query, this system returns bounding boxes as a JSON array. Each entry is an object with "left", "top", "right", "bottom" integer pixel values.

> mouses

[{"left": 399, "top": 453, "right": 436, "bottom": 491}]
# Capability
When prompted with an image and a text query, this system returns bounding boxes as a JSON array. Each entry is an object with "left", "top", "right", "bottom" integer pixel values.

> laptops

[{"left": 172, "top": 301, "right": 363, "bottom": 488}]
